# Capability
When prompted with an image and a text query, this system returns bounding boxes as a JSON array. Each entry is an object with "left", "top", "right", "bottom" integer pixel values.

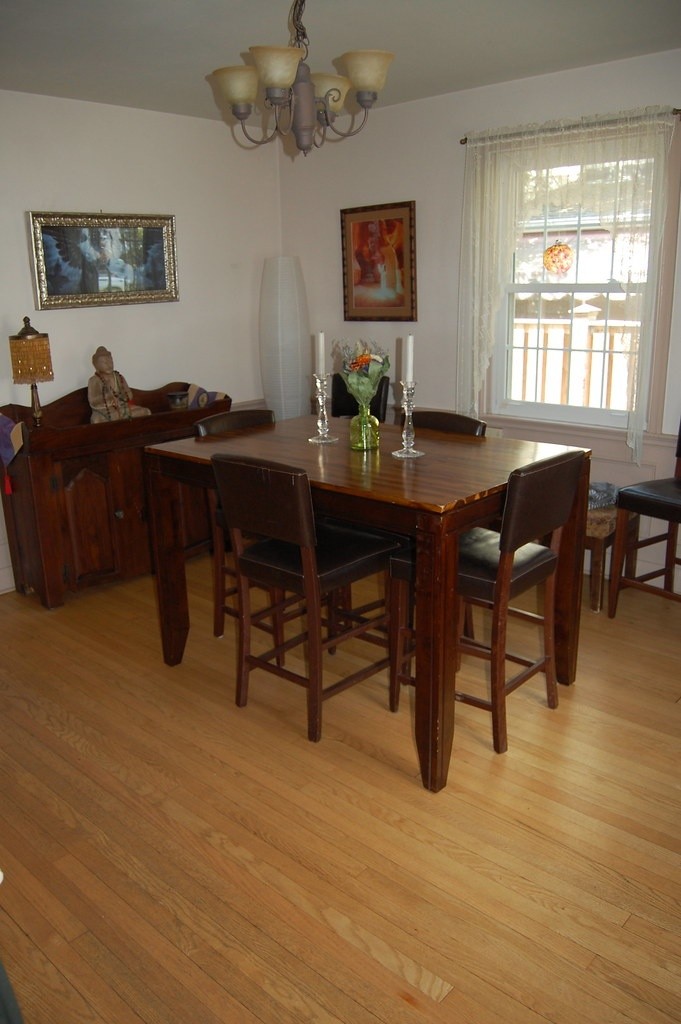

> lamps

[
  {"left": 204, "top": 0, "right": 393, "bottom": 157},
  {"left": 8, "top": 315, "right": 54, "bottom": 427}
]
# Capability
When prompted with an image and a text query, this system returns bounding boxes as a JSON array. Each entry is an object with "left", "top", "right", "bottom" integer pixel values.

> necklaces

[{"left": 95, "top": 371, "right": 132, "bottom": 420}]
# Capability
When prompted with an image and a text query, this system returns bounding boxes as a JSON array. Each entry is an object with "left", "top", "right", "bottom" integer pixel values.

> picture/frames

[
  {"left": 27, "top": 210, "right": 180, "bottom": 310},
  {"left": 340, "top": 200, "right": 418, "bottom": 322}
]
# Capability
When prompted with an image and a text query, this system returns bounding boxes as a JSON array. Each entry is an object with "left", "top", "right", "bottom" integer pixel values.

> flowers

[{"left": 333, "top": 336, "right": 391, "bottom": 404}]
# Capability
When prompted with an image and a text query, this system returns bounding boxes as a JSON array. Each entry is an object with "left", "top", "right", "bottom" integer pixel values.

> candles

[
  {"left": 405, "top": 334, "right": 414, "bottom": 387},
  {"left": 317, "top": 331, "right": 325, "bottom": 375}
]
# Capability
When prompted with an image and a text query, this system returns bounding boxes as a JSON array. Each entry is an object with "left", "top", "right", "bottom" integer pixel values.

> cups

[{"left": 167, "top": 392, "right": 189, "bottom": 410}]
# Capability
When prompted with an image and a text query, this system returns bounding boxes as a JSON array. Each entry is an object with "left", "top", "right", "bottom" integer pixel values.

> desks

[{"left": 146, "top": 414, "right": 593, "bottom": 792}]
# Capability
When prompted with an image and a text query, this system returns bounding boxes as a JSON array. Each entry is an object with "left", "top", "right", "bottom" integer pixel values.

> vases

[{"left": 349, "top": 405, "right": 379, "bottom": 450}]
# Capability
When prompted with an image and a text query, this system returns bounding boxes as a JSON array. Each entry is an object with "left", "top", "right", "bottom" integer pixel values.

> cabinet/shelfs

[{"left": 0, "top": 381, "right": 232, "bottom": 610}]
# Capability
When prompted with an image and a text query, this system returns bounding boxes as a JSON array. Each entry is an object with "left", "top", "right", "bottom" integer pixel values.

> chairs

[
  {"left": 398, "top": 408, "right": 487, "bottom": 632},
  {"left": 195, "top": 409, "right": 352, "bottom": 667},
  {"left": 607, "top": 423, "right": 681, "bottom": 618},
  {"left": 209, "top": 452, "right": 401, "bottom": 742},
  {"left": 388, "top": 450, "right": 585, "bottom": 753}
]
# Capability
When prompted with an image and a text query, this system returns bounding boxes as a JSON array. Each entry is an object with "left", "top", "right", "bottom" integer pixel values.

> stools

[{"left": 587, "top": 506, "right": 640, "bottom": 613}]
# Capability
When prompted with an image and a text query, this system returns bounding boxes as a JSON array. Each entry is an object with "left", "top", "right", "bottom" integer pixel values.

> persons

[{"left": 88, "top": 345, "right": 151, "bottom": 424}]
